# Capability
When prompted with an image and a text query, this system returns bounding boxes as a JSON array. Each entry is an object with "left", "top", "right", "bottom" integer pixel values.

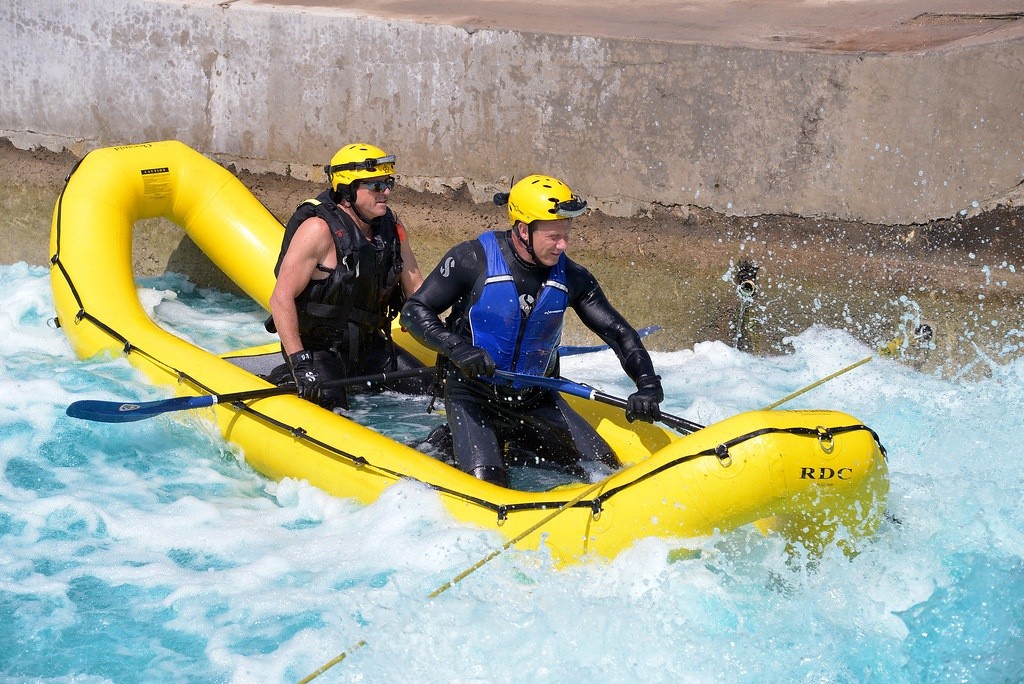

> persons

[
  {"left": 399, "top": 174, "right": 664, "bottom": 485},
  {"left": 265, "top": 143, "right": 444, "bottom": 411}
]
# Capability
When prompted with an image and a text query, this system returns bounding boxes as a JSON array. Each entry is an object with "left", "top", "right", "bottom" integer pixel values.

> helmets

[
  {"left": 508, "top": 174, "right": 587, "bottom": 226},
  {"left": 330, "top": 143, "right": 398, "bottom": 192}
]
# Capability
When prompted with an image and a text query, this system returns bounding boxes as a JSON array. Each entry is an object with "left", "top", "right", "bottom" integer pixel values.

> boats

[{"left": 45, "top": 138, "right": 890, "bottom": 570}]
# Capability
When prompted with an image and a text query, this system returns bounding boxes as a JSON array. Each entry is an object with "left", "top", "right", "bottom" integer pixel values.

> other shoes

[{"left": 406, "top": 422, "right": 451, "bottom": 460}]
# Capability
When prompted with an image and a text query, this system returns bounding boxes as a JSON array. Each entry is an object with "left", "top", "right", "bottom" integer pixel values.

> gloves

[
  {"left": 440, "top": 334, "right": 496, "bottom": 379},
  {"left": 288, "top": 349, "right": 324, "bottom": 404},
  {"left": 625, "top": 386, "right": 664, "bottom": 423}
]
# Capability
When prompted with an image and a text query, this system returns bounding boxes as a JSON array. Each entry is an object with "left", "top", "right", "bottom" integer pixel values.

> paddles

[
  {"left": 67, "top": 324, "right": 662, "bottom": 423},
  {"left": 486, "top": 369, "right": 707, "bottom": 432}
]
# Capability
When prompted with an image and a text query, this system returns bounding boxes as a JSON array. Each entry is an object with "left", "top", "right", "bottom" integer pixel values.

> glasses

[{"left": 356, "top": 176, "right": 395, "bottom": 192}]
